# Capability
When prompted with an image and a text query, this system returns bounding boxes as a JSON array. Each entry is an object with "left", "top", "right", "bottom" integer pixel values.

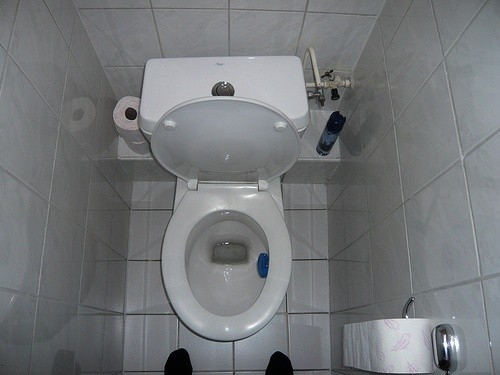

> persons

[{"left": 163, "top": 348, "right": 293, "bottom": 375}]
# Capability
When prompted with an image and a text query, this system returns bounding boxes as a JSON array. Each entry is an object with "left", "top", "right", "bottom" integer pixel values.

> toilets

[{"left": 137, "top": 54, "right": 311, "bottom": 341}]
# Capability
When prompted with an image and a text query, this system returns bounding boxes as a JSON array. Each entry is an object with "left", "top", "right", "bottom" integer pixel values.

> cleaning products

[{"left": 315, "top": 110, "right": 347, "bottom": 156}]
